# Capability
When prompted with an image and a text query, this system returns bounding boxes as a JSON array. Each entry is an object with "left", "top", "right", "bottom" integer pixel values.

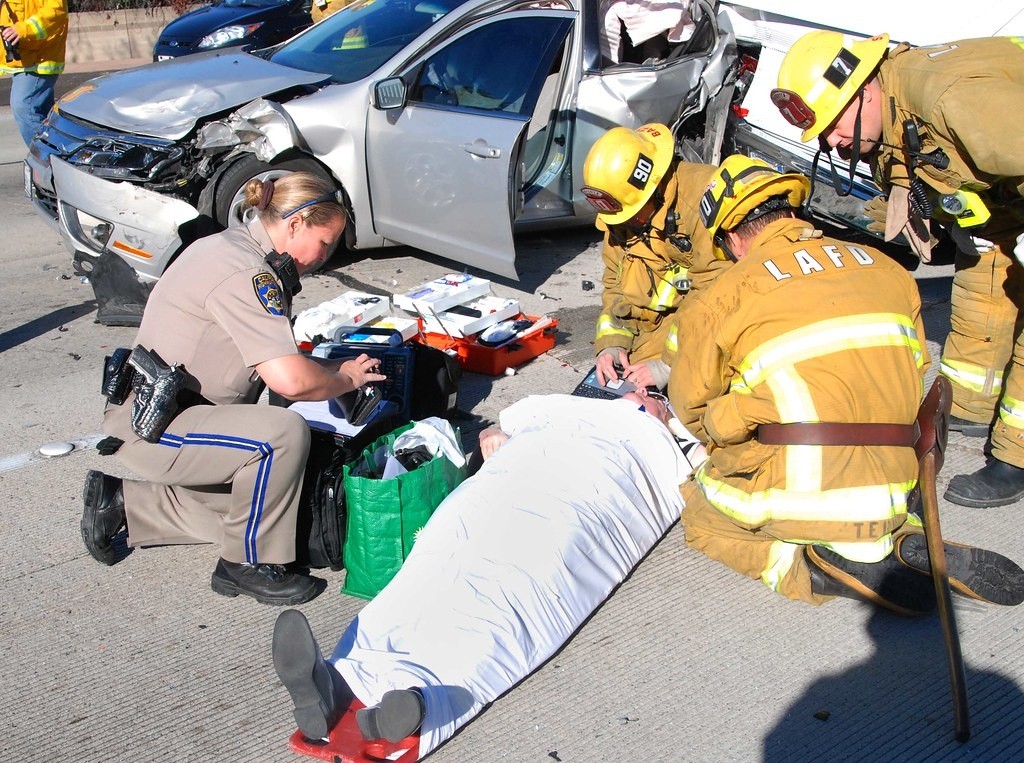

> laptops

[{"left": 570, "top": 363, "right": 667, "bottom": 401}]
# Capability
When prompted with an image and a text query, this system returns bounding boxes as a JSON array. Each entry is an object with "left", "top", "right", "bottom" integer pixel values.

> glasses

[
  {"left": 647, "top": 393, "right": 665, "bottom": 401},
  {"left": 281, "top": 189, "right": 349, "bottom": 219}
]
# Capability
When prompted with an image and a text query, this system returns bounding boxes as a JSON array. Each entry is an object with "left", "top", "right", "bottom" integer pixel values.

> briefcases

[
  {"left": 312, "top": 326, "right": 410, "bottom": 400},
  {"left": 410, "top": 310, "right": 560, "bottom": 375}
]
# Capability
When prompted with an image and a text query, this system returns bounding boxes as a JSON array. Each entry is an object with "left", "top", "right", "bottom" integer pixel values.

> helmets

[
  {"left": 699, "top": 154, "right": 812, "bottom": 248},
  {"left": 770, "top": 30, "right": 889, "bottom": 142},
  {"left": 582, "top": 122, "right": 675, "bottom": 226}
]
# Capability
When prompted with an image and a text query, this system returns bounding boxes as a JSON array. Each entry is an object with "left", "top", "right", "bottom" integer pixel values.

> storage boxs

[{"left": 280, "top": 267, "right": 556, "bottom": 457}]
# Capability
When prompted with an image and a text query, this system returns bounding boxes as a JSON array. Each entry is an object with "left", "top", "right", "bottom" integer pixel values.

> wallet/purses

[{"left": 334, "top": 382, "right": 383, "bottom": 426}]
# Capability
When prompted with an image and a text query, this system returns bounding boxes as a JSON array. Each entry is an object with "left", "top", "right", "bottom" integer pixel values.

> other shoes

[
  {"left": 892, "top": 532, "right": 1024, "bottom": 606},
  {"left": 803, "top": 545, "right": 939, "bottom": 612}
]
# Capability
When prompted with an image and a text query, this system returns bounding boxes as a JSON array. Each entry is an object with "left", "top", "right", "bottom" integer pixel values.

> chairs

[{"left": 380, "top": 16, "right": 536, "bottom": 115}]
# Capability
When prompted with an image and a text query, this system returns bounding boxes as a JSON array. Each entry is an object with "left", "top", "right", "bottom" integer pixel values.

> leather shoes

[
  {"left": 944, "top": 461, "right": 1024, "bottom": 508},
  {"left": 355, "top": 685, "right": 426, "bottom": 745},
  {"left": 81, "top": 470, "right": 124, "bottom": 567},
  {"left": 945, "top": 413, "right": 994, "bottom": 439},
  {"left": 271, "top": 610, "right": 336, "bottom": 741},
  {"left": 212, "top": 558, "right": 316, "bottom": 606}
]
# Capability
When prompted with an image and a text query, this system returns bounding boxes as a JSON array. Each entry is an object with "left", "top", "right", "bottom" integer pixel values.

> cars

[
  {"left": 22, "top": 0, "right": 741, "bottom": 286},
  {"left": 155, "top": 0, "right": 314, "bottom": 64}
]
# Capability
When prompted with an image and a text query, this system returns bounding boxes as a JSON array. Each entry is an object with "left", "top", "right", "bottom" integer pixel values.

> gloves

[{"left": 865, "top": 194, "right": 939, "bottom": 252}]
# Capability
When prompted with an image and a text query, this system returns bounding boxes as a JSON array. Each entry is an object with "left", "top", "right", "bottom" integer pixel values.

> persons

[
  {"left": 667, "top": 154, "right": 1024, "bottom": 618},
  {"left": 0, "top": 0, "right": 68, "bottom": 152},
  {"left": 81, "top": 171, "right": 387, "bottom": 607},
  {"left": 580, "top": 123, "right": 733, "bottom": 390},
  {"left": 769, "top": 28, "right": 1024, "bottom": 509},
  {"left": 311, "top": 0, "right": 376, "bottom": 51},
  {"left": 271, "top": 387, "right": 675, "bottom": 742}
]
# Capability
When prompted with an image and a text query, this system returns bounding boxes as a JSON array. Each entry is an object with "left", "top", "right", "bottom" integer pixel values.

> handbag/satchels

[
  {"left": 308, "top": 417, "right": 468, "bottom": 603},
  {"left": 407, "top": 337, "right": 462, "bottom": 417}
]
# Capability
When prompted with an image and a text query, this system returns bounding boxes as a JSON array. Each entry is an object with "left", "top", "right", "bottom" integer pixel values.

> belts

[{"left": 754, "top": 424, "right": 920, "bottom": 446}]
